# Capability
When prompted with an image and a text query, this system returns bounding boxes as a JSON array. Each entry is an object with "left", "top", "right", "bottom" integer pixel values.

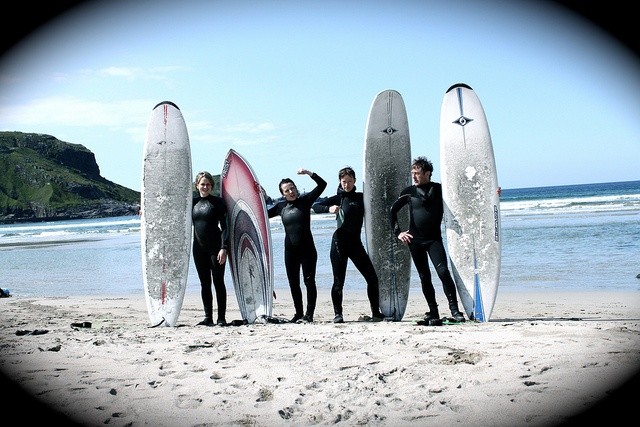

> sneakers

[
  {"left": 451, "top": 311, "right": 466, "bottom": 322},
  {"left": 333, "top": 313, "right": 343, "bottom": 323},
  {"left": 424, "top": 311, "right": 440, "bottom": 320},
  {"left": 290, "top": 313, "right": 304, "bottom": 322},
  {"left": 371, "top": 313, "right": 385, "bottom": 321},
  {"left": 296, "top": 315, "right": 314, "bottom": 324},
  {"left": 217, "top": 318, "right": 227, "bottom": 326},
  {"left": 198, "top": 319, "right": 214, "bottom": 326}
]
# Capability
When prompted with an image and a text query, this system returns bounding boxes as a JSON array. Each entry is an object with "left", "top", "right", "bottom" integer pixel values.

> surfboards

[
  {"left": 438, "top": 82, "right": 501, "bottom": 323},
  {"left": 219, "top": 149, "right": 276, "bottom": 323},
  {"left": 363, "top": 89, "right": 411, "bottom": 322},
  {"left": 140, "top": 101, "right": 192, "bottom": 326}
]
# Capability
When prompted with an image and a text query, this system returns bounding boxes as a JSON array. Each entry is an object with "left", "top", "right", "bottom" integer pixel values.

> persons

[
  {"left": 267, "top": 169, "right": 327, "bottom": 323},
  {"left": 390, "top": 157, "right": 500, "bottom": 322},
  {"left": 139, "top": 249, "right": 189, "bottom": 250},
  {"left": 312, "top": 168, "right": 385, "bottom": 323},
  {"left": 191, "top": 171, "right": 230, "bottom": 327}
]
